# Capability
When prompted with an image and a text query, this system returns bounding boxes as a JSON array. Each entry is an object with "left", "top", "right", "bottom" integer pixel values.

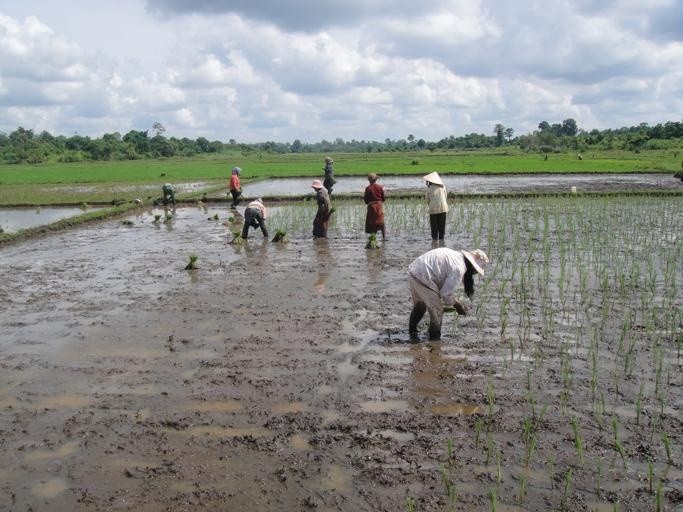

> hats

[
  {"left": 310, "top": 179, "right": 322, "bottom": 189},
  {"left": 459, "top": 246, "right": 489, "bottom": 277},
  {"left": 421, "top": 171, "right": 444, "bottom": 185}
]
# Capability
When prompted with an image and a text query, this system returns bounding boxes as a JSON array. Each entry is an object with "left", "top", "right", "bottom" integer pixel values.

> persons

[
  {"left": 365, "top": 241, "right": 387, "bottom": 284},
  {"left": 242, "top": 198, "right": 269, "bottom": 239},
  {"left": 163, "top": 206, "right": 176, "bottom": 232},
  {"left": 422, "top": 171, "right": 449, "bottom": 239},
  {"left": 312, "top": 180, "right": 332, "bottom": 237},
  {"left": 410, "top": 344, "right": 478, "bottom": 416},
  {"left": 408, "top": 247, "right": 489, "bottom": 340},
  {"left": 229, "top": 209, "right": 243, "bottom": 234},
  {"left": 243, "top": 239, "right": 268, "bottom": 268},
  {"left": 322, "top": 157, "right": 334, "bottom": 194},
  {"left": 577, "top": 153, "right": 582, "bottom": 160},
  {"left": 163, "top": 182, "right": 176, "bottom": 205},
  {"left": 364, "top": 173, "right": 386, "bottom": 239},
  {"left": 229, "top": 167, "right": 242, "bottom": 209},
  {"left": 314, "top": 239, "right": 336, "bottom": 292}
]
